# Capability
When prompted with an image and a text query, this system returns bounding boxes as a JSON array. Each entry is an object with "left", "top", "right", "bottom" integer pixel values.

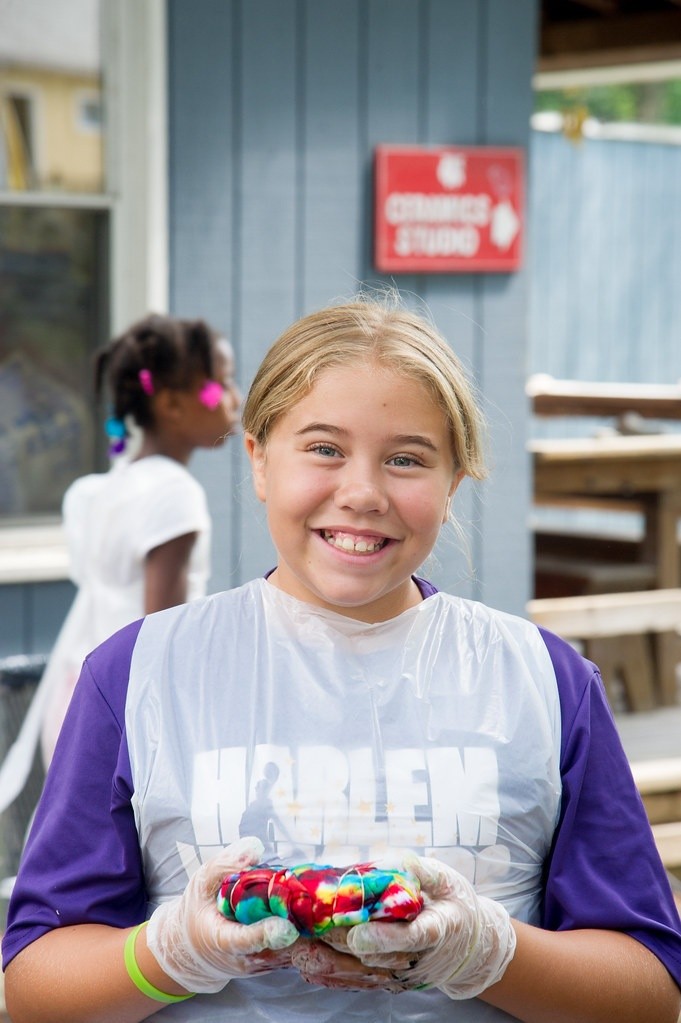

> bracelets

[{"left": 125, "top": 922, "right": 197, "bottom": 1003}]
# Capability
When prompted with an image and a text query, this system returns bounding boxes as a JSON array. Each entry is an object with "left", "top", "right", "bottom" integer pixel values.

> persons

[
  {"left": 38, "top": 314, "right": 237, "bottom": 774},
  {"left": 4, "top": 302, "right": 681, "bottom": 1023}
]
